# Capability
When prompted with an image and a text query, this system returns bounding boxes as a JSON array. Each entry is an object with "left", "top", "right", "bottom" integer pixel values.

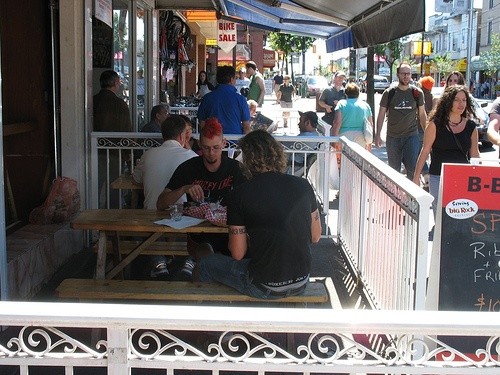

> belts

[{"left": 252, "top": 276, "right": 310, "bottom": 296}]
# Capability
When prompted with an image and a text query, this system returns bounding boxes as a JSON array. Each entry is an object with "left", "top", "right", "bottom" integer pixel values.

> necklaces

[{"left": 447, "top": 115, "right": 462, "bottom": 127}]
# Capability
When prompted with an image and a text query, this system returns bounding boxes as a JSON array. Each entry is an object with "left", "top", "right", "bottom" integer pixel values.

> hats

[{"left": 299, "top": 109, "right": 319, "bottom": 127}]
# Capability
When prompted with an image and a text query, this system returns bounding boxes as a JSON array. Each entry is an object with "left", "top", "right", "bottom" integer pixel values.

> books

[{"left": 250, "top": 111, "right": 279, "bottom": 135}]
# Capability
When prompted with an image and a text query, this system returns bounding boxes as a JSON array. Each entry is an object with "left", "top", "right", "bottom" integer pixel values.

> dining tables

[{"left": 68, "top": 208, "right": 251, "bottom": 281}]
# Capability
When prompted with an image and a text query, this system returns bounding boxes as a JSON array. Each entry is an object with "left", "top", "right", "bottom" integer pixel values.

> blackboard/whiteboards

[{"left": 427, "top": 206, "right": 500, "bottom": 356}]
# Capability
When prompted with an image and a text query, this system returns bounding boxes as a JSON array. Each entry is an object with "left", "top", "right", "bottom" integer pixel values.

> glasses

[
  {"left": 398, "top": 71, "right": 410, "bottom": 75},
  {"left": 201, "top": 145, "right": 220, "bottom": 152}
]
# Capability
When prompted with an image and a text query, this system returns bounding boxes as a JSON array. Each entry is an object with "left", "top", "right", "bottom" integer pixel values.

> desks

[{"left": 111, "top": 174, "right": 144, "bottom": 208}]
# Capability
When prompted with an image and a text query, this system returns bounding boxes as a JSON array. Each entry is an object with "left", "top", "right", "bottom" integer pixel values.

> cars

[
  {"left": 293, "top": 74, "right": 389, "bottom": 99},
  {"left": 418, "top": 87, "right": 493, "bottom": 148}
]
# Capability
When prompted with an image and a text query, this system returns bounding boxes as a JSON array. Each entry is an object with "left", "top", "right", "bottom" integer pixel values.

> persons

[
  {"left": 418, "top": 75, "right": 437, "bottom": 174},
  {"left": 246, "top": 98, "right": 278, "bottom": 134},
  {"left": 273, "top": 70, "right": 283, "bottom": 104},
  {"left": 196, "top": 128, "right": 322, "bottom": 355},
  {"left": 156, "top": 117, "right": 255, "bottom": 263},
  {"left": 178, "top": 114, "right": 200, "bottom": 153},
  {"left": 246, "top": 61, "right": 266, "bottom": 107},
  {"left": 332, "top": 82, "right": 373, "bottom": 200},
  {"left": 136, "top": 102, "right": 169, "bottom": 160},
  {"left": 196, "top": 65, "right": 251, "bottom": 157},
  {"left": 375, "top": 62, "right": 428, "bottom": 182},
  {"left": 318, "top": 70, "right": 347, "bottom": 125},
  {"left": 131, "top": 113, "right": 199, "bottom": 279},
  {"left": 276, "top": 75, "right": 295, "bottom": 128},
  {"left": 136, "top": 69, "right": 145, "bottom": 106},
  {"left": 487, "top": 96, "right": 500, "bottom": 157},
  {"left": 195, "top": 70, "right": 215, "bottom": 99},
  {"left": 444, "top": 70, "right": 467, "bottom": 90},
  {"left": 285, "top": 109, "right": 320, "bottom": 174},
  {"left": 234, "top": 66, "right": 252, "bottom": 95},
  {"left": 92, "top": 69, "right": 134, "bottom": 209},
  {"left": 412, "top": 83, "right": 482, "bottom": 225}
]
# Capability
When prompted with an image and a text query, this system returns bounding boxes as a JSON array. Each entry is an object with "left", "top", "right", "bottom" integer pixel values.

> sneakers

[
  {"left": 150, "top": 262, "right": 171, "bottom": 280},
  {"left": 180, "top": 259, "right": 196, "bottom": 278}
]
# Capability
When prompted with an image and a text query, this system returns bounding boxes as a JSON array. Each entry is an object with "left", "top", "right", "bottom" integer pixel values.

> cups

[{"left": 168, "top": 203, "right": 183, "bottom": 221}]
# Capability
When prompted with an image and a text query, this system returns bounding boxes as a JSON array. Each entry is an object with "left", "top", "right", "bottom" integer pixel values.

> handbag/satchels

[{"left": 28, "top": 177, "right": 80, "bottom": 225}]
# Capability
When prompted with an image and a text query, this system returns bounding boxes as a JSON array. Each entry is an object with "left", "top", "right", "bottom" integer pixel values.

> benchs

[{"left": 53, "top": 278, "right": 329, "bottom": 356}]
